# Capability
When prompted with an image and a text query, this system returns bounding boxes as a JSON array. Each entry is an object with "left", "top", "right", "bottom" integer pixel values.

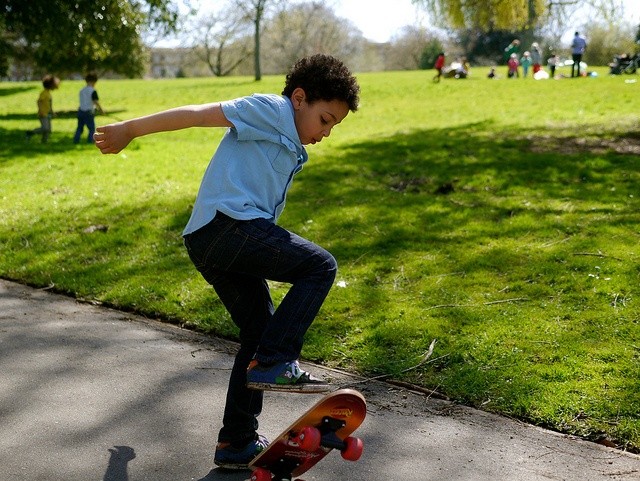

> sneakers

[
  {"left": 245, "top": 346, "right": 329, "bottom": 392},
  {"left": 214, "top": 434, "right": 270, "bottom": 469}
]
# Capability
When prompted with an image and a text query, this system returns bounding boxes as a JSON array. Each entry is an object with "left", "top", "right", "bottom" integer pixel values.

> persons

[
  {"left": 74, "top": 72, "right": 104, "bottom": 143},
  {"left": 93, "top": 53, "right": 359, "bottom": 470},
  {"left": 508, "top": 53, "right": 518, "bottom": 77},
  {"left": 455, "top": 55, "right": 469, "bottom": 75},
  {"left": 504, "top": 39, "right": 521, "bottom": 77},
  {"left": 571, "top": 31, "right": 587, "bottom": 76},
  {"left": 25, "top": 74, "right": 60, "bottom": 144},
  {"left": 432, "top": 52, "right": 444, "bottom": 81},
  {"left": 547, "top": 54, "right": 557, "bottom": 78},
  {"left": 531, "top": 43, "right": 542, "bottom": 73},
  {"left": 488, "top": 68, "right": 496, "bottom": 77},
  {"left": 520, "top": 51, "right": 532, "bottom": 76}
]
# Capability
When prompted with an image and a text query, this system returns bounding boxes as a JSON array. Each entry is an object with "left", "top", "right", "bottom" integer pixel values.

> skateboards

[{"left": 248, "top": 387, "right": 368, "bottom": 480}]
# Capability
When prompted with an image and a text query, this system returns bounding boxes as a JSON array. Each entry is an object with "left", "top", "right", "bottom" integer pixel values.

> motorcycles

[{"left": 609, "top": 54, "right": 638, "bottom": 74}]
